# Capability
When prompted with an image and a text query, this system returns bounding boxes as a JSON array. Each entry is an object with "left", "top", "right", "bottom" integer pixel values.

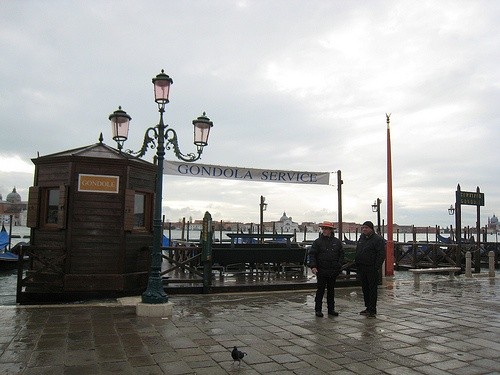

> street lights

[
  {"left": 372, "top": 198, "right": 382, "bottom": 237},
  {"left": 448, "top": 205, "right": 457, "bottom": 239},
  {"left": 108, "top": 69, "right": 213, "bottom": 304},
  {"left": 260, "top": 195, "right": 267, "bottom": 243}
]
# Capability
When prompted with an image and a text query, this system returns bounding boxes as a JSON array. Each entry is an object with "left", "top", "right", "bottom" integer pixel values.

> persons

[
  {"left": 354, "top": 220, "right": 385, "bottom": 318},
  {"left": 309, "top": 221, "right": 344, "bottom": 317}
]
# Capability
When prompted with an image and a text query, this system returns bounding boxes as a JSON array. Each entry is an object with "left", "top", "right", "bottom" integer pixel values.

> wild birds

[{"left": 231, "top": 346, "right": 247, "bottom": 367}]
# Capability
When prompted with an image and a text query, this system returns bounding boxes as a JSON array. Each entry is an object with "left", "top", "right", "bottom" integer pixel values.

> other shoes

[
  {"left": 360, "top": 310, "right": 370, "bottom": 314},
  {"left": 367, "top": 315, "right": 376, "bottom": 319},
  {"left": 328, "top": 312, "right": 338, "bottom": 316},
  {"left": 315, "top": 312, "right": 324, "bottom": 317}
]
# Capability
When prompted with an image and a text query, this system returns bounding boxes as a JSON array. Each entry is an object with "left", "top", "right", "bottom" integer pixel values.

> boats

[
  {"left": 233, "top": 237, "right": 258, "bottom": 244},
  {"left": 264, "top": 237, "right": 288, "bottom": 244},
  {"left": 171, "top": 233, "right": 307, "bottom": 270},
  {"left": 0, "top": 221, "right": 30, "bottom": 271}
]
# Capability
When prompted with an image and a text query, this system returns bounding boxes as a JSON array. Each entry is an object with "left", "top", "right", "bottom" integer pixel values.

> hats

[
  {"left": 362, "top": 221, "right": 374, "bottom": 231},
  {"left": 319, "top": 220, "right": 336, "bottom": 230}
]
